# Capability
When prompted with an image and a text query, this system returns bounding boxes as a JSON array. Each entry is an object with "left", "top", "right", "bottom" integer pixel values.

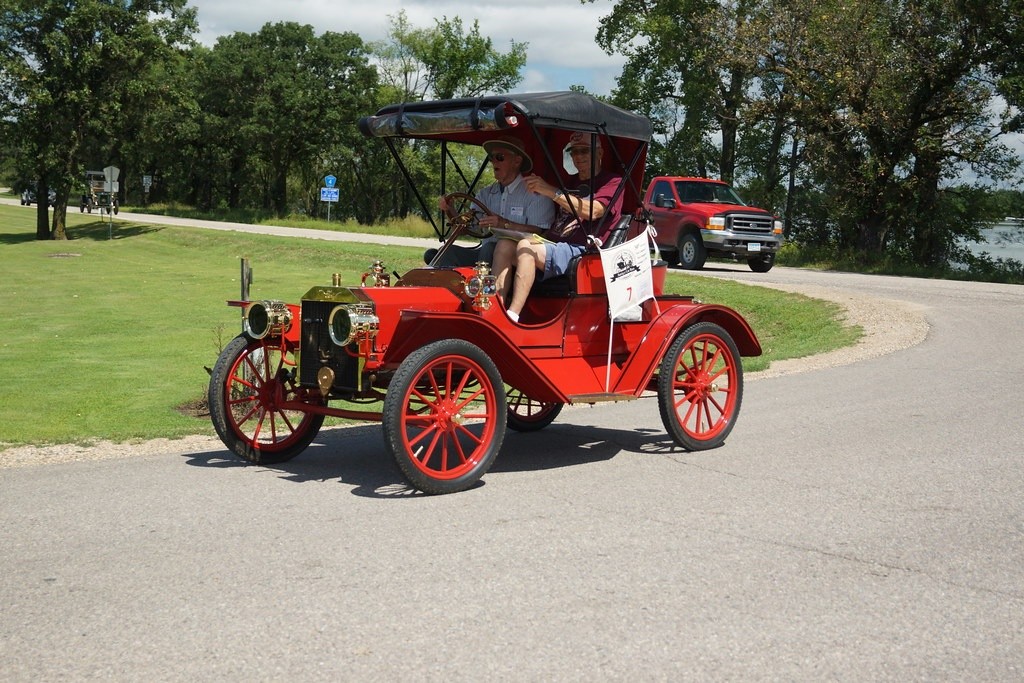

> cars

[
  {"left": 207, "top": 92, "right": 762, "bottom": 496},
  {"left": 20, "top": 180, "right": 57, "bottom": 207}
]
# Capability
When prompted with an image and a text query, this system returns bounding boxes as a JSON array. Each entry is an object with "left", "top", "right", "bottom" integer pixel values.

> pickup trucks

[{"left": 626, "top": 175, "right": 784, "bottom": 273}]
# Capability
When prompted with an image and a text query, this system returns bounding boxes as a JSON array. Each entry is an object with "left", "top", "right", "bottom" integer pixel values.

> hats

[
  {"left": 566, "top": 131, "right": 601, "bottom": 152},
  {"left": 482, "top": 135, "right": 533, "bottom": 173}
]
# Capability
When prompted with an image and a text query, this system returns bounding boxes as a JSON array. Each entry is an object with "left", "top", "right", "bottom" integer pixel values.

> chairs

[{"left": 691, "top": 187, "right": 714, "bottom": 202}]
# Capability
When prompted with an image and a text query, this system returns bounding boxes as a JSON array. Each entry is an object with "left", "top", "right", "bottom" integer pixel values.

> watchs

[
  {"left": 552, "top": 188, "right": 562, "bottom": 201},
  {"left": 504, "top": 219, "right": 510, "bottom": 229}
]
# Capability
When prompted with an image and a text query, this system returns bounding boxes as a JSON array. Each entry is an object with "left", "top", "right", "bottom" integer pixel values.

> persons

[
  {"left": 424, "top": 135, "right": 556, "bottom": 276},
  {"left": 699, "top": 187, "right": 713, "bottom": 200},
  {"left": 491, "top": 130, "right": 625, "bottom": 322}
]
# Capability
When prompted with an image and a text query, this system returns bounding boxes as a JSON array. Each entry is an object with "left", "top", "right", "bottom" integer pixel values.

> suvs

[{"left": 80, "top": 170, "right": 119, "bottom": 215}]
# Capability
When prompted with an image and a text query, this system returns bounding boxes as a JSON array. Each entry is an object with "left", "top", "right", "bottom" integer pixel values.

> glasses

[
  {"left": 569, "top": 148, "right": 591, "bottom": 156},
  {"left": 487, "top": 154, "right": 515, "bottom": 162}
]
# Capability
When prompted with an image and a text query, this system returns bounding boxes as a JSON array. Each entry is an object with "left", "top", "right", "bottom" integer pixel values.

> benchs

[{"left": 433, "top": 214, "right": 634, "bottom": 298}]
